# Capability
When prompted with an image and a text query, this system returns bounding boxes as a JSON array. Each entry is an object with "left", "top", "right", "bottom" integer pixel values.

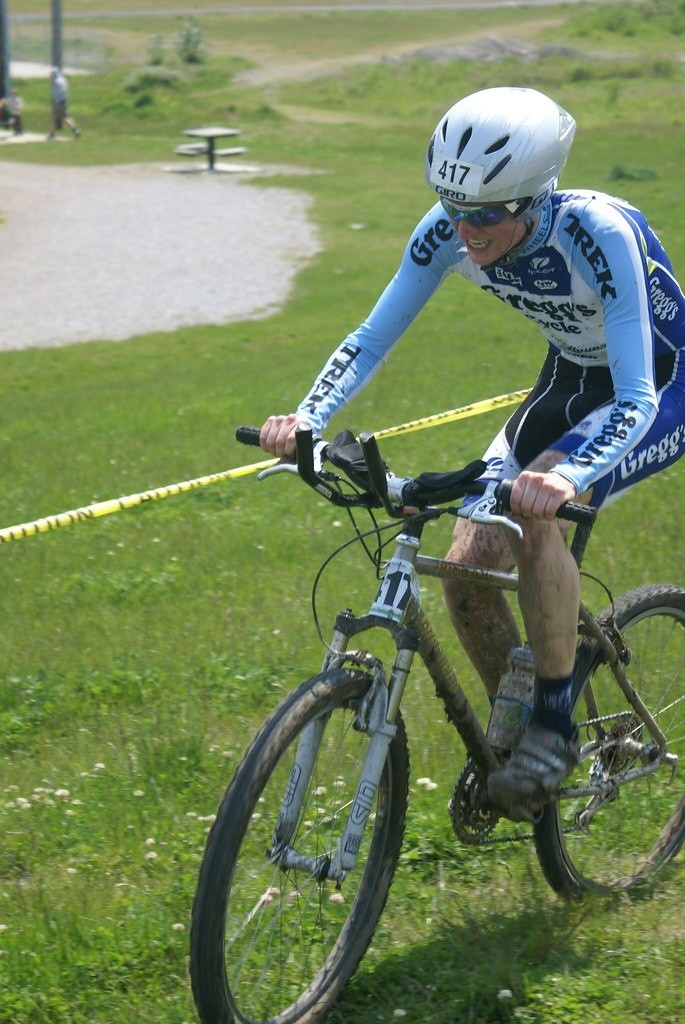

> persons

[
  {"left": 48, "top": 69, "right": 81, "bottom": 140},
  {"left": 0, "top": 91, "right": 22, "bottom": 134},
  {"left": 260, "top": 87, "right": 685, "bottom": 806}
]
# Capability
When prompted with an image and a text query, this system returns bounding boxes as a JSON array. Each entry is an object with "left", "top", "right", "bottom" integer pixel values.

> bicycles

[{"left": 189, "top": 429, "right": 684, "bottom": 1023}]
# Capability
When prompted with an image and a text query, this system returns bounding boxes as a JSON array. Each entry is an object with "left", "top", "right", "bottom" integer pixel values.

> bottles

[{"left": 487, "top": 641, "right": 536, "bottom": 751}]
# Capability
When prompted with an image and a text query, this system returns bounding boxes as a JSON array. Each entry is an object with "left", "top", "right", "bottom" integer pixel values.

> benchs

[{"left": 174, "top": 143, "right": 245, "bottom": 156}]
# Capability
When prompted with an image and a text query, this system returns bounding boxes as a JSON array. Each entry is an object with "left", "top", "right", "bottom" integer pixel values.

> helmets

[{"left": 425, "top": 87, "right": 576, "bottom": 201}]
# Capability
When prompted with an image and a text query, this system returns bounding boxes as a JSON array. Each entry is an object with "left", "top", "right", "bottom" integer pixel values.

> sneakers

[
  {"left": 487, "top": 722, "right": 581, "bottom": 809},
  {"left": 446, "top": 772, "right": 490, "bottom": 825}
]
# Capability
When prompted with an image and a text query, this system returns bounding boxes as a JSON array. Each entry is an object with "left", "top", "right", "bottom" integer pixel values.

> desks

[{"left": 181, "top": 128, "right": 240, "bottom": 171}]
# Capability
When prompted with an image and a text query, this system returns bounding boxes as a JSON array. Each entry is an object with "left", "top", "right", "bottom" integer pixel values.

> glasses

[{"left": 439, "top": 197, "right": 514, "bottom": 229}]
